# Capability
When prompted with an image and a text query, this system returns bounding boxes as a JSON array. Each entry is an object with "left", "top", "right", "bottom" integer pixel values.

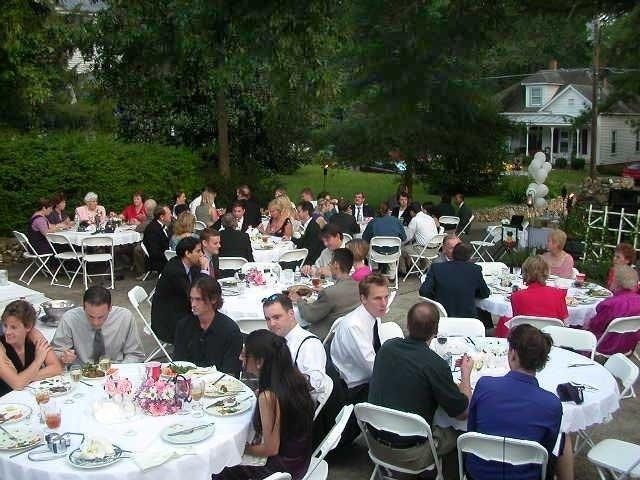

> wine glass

[
  {"left": 32, "top": 352, "right": 206, "bottom": 437},
  {"left": 501, "top": 266, "right": 522, "bottom": 283}
]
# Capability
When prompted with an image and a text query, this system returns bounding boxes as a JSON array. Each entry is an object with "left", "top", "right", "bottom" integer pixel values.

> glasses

[{"left": 262, "top": 295, "right": 278, "bottom": 302}]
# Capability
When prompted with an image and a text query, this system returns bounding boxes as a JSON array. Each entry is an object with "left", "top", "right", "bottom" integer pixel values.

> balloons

[{"left": 525, "top": 151, "right": 552, "bottom": 209}]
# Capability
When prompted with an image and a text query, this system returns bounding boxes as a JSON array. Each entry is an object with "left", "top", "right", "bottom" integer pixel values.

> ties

[
  {"left": 374, "top": 320, "right": 382, "bottom": 353},
  {"left": 95, "top": 331, "right": 104, "bottom": 358}
]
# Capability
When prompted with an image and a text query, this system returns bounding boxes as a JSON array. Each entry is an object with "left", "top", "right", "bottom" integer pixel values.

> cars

[
  {"left": 622, "top": 161, "right": 640, "bottom": 184},
  {"left": 357, "top": 157, "right": 408, "bottom": 174}
]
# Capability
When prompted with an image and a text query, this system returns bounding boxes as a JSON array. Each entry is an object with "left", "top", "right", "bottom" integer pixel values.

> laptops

[{"left": 502, "top": 215, "right": 524, "bottom": 228}]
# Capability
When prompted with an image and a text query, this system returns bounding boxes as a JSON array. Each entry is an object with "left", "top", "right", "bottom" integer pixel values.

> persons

[
  {"left": 29, "top": 191, "right": 157, "bottom": 280},
  {"left": 329, "top": 274, "right": 389, "bottom": 404},
  {"left": 0, "top": 300, "right": 62, "bottom": 398},
  {"left": 466, "top": 323, "right": 574, "bottom": 480},
  {"left": 262, "top": 293, "right": 327, "bottom": 406},
  {"left": 143, "top": 187, "right": 472, "bottom": 344},
  {"left": 211, "top": 329, "right": 315, "bottom": 479},
  {"left": 50, "top": 285, "right": 145, "bottom": 363},
  {"left": 172, "top": 275, "right": 243, "bottom": 380},
  {"left": 365, "top": 301, "right": 475, "bottom": 471},
  {"left": 419, "top": 230, "right": 640, "bottom": 356}
]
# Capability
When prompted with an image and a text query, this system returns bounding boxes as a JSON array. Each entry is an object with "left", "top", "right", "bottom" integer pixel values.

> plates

[
  {"left": 484, "top": 272, "right": 612, "bottom": 306},
  {"left": 286, "top": 278, "right": 335, "bottom": 306},
  {"left": 0, "top": 371, "right": 253, "bottom": 468}
]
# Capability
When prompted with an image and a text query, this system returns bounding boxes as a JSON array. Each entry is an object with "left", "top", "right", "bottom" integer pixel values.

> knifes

[{"left": 568, "top": 363, "right": 596, "bottom": 367}]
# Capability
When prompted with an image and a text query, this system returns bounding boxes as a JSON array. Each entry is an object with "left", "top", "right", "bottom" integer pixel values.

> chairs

[
  {"left": 506, "top": 316, "right": 640, "bottom": 480},
  {"left": 312, "top": 374, "right": 334, "bottom": 421},
  {"left": 354, "top": 402, "right": 445, "bottom": 480},
  {"left": 456, "top": 432, "right": 548, "bottom": 480},
  {"left": 297, "top": 404, "right": 355, "bottom": 480}
]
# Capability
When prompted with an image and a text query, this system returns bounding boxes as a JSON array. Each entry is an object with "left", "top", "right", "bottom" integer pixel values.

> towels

[{"left": 129, "top": 444, "right": 198, "bottom": 472}]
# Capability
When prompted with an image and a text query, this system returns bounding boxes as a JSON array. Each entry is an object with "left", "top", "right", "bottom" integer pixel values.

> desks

[{"left": 0, "top": 279, "right": 77, "bottom": 347}]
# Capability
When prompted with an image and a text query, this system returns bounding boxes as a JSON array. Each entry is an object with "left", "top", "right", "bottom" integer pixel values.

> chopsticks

[{"left": 467, "top": 336, "right": 487, "bottom": 352}]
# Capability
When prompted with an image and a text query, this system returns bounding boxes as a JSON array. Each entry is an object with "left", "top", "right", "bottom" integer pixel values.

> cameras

[
  {"left": 557, "top": 383, "right": 584, "bottom": 404},
  {"left": 105, "top": 225, "right": 116, "bottom": 232}
]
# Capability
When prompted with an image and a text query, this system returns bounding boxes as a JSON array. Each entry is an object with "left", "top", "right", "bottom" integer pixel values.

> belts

[
  {"left": 373, "top": 437, "right": 421, "bottom": 449},
  {"left": 422, "top": 246, "right": 439, "bottom": 249}
]
